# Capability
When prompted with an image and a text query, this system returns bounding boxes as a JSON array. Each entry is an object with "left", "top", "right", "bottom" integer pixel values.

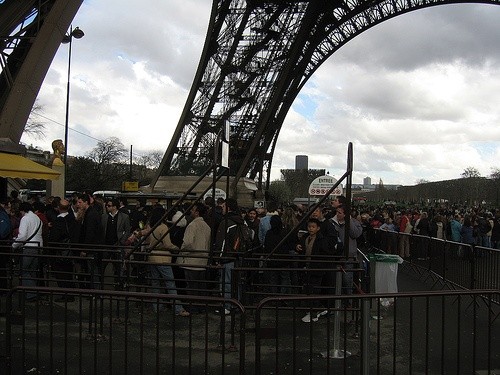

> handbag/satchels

[
  {"left": 147, "top": 247, "right": 172, "bottom": 265},
  {"left": 10, "top": 248, "right": 21, "bottom": 265}
]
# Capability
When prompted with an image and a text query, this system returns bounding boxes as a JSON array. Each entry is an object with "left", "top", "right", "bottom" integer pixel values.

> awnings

[{"left": 0, "top": 153, "right": 62, "bottom": 182}]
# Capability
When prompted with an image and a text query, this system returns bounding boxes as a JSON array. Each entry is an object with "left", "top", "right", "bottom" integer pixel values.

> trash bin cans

[{"left": 368, "top": 253, "right": 400, "bottom": 302}]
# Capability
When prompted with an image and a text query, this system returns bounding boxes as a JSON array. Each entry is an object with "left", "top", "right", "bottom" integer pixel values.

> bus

[{"left": 18, "top": 189, "right": 80, "bottom": 201}]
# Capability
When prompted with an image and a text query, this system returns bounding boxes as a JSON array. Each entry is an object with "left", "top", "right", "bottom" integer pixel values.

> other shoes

[
  {"left": 54, "top": 297, "right": 75, "bottom": 302},
  {"left": 215, "top": 308, "right": 230, "bottom": 314},
  {"left": 301, "top": 310, "right": 327, "bottom": 322},
  {"left": 22, "top": 300, "right": 41, "bottom": 307},
  {"left": 177, "top": 311, "right": 190, "bottom": 316}
]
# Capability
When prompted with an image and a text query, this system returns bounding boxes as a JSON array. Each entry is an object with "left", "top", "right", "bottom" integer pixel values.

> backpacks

[
  {"left": 233, "top": 224, "right": 253, "bottom": 252},
  {"left": 0, "top": 211, "right": 10, "bottom": 238},
  {"left": 404, "top": 222, "right": 413, "bottom": 233}
]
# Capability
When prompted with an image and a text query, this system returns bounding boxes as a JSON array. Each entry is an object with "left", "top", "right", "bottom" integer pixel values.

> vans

[{"left": 93, "top": 191, "right": 121, "bottom": 199}]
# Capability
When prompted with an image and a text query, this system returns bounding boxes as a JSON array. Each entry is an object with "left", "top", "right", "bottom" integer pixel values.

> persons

[
  {"left": 351, "top": 203, "right": 500, "bottom": 283},
  {"left": 323, "top": 196, "right": 362, "bottom": 307},
  {"left": 0, "top": 190, "right": 185, "bottom": 313},
  {"left": 176, "top": 196, "right": 339, "bottom": 322},
  {"left": 49, "top": 139, "right": 65, "bottom": 165}
]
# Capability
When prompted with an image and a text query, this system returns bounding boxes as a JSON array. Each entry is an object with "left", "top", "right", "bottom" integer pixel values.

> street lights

[{"left": 61, "top": 21, "right": 86, "bottom": 171}]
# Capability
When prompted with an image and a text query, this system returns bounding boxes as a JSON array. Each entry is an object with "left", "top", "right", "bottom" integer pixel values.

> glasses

[{"left": 106, "top": 205, "right": 113, "bottom": 207}]
